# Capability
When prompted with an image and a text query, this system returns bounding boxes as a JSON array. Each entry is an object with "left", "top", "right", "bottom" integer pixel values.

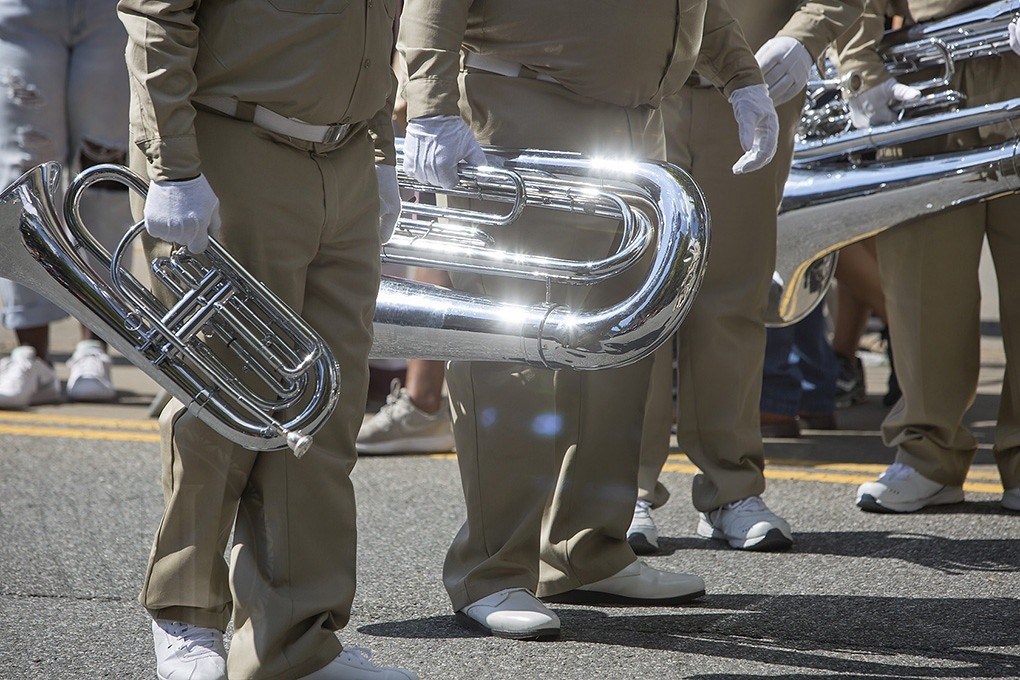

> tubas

[
  {"left": 762, "top": 0, "right": 1020, "bottom": 333},
  {"left": 0, "top": 160, "right": 343, "bottom": 459},
  {"left": 367, "top": 136, "right": 713, "bottom": 371}
]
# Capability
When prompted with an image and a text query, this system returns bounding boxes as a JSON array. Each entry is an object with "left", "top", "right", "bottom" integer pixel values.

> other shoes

[{"left": 759, "top": 408, "right": 838, "bottom": 438}]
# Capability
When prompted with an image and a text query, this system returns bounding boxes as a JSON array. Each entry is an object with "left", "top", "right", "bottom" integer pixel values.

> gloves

[
  {"left": 847, "top": 75, "right": 922, "bottom": 130},
  {"left": 375, "top": 164, "right": 404, "bottom": 244},
  {"left": 143, "top": 172, "right": 222, "bottom": 255},
  {"left": 1008, "top": 17, "right": 1020, "bottom": 57},
  {"left": 753, "top": 35, "right": 814, "bottom": 108},
  {"left": 727, "top": 83, "right": 780, "bottom": 175},
  {"left": 402, "top": 115, "right": 488, "bottom": 189}
]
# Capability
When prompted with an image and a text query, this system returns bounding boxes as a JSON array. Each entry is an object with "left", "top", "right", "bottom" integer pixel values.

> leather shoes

[
  {"left": 458, "top": 587, "right": 562, "bottom": 639},
  {"left": 539, "top": 558, "right": 707, "bottom": 607}
]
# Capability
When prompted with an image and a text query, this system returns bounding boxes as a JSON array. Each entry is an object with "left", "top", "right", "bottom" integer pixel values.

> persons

[
  {"left": 621, "top": 1, "right": 868, "bottom": 556},
  {"left": 393, "top": 1, "right": 781, "bottom": 638},
  {"left": 117, "top": 0, "right": 412, "bottom": 680},
  {"left": 0, "top": 0, "right": 130, "bottom": 408},
  {"left": 836, "top": 2, "right": 1020, "bottom": 513},
  {"left": 354, "top": 28, "right": 902, "bottom": 456}
]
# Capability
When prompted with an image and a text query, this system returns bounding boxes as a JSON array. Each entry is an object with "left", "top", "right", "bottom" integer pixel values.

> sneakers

[
  {"left": 65, "top": 339, "right": 116, "bottom": 402},
  {"left": 828, "top": 349, "right": 866, "bottom": 408},
  {"left": 0, "top": 345, "right": 63, "bottom": 409},
  {"left": 296, "top": 645, "right": 420, "bottom": 679},
  {"left": 855, "top": 460, "right": 966, "bottom": 513},
  {"left": 697, "top": 495, "right": 794, "bottom": 552},
  {"left": 355, "top": 388, "right": 455, "bottom": 455},
  {"left": 881, "top": 325, "right": 903, "bottom": 407},
  {"left": 152, "top": 619, "right": 228, "bottom": 680},
  {"left": 626, "top": 499, "right": 660, "bottom": 555}
]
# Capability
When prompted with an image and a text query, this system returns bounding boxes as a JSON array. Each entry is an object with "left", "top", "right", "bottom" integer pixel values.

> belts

[
  {"left": 190, "top": 90, "right": 351, "bottom": 145},
  {"left": 464, "top": 51, "right": 562, "bottom": 85}
]
